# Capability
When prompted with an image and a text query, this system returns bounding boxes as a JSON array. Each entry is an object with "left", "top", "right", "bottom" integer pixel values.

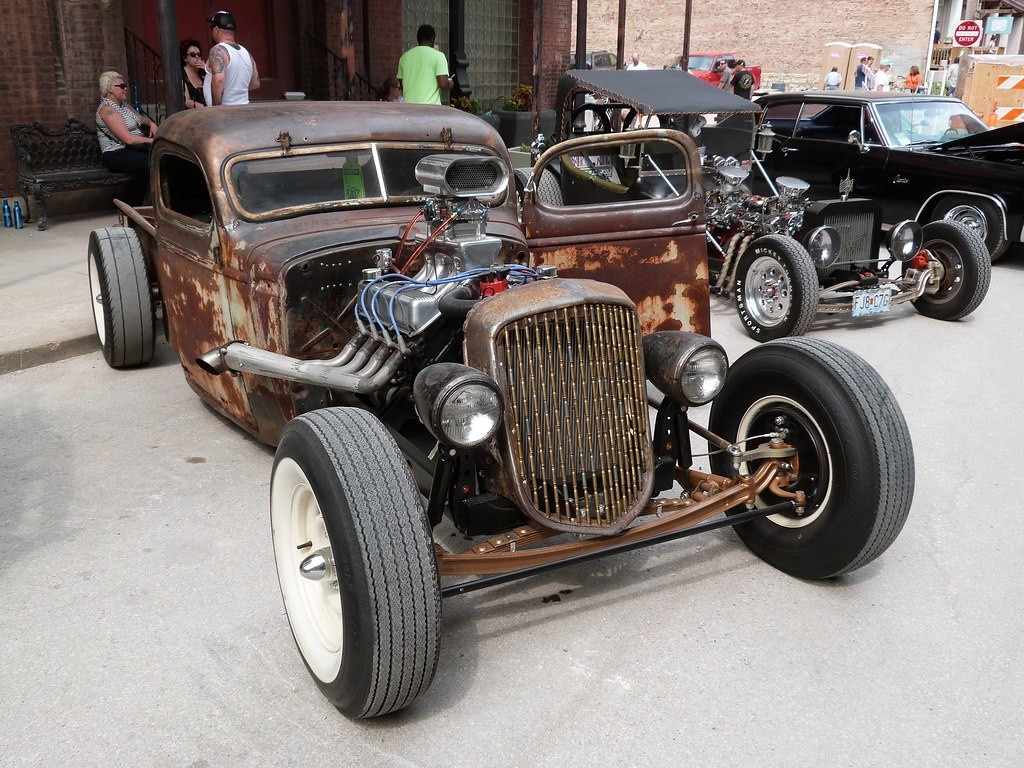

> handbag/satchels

[{"left": 126, "top": 142, "right": 151, "bottom": 153}]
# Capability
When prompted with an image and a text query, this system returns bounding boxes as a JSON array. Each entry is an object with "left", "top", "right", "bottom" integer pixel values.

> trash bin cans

[
  {"left": 492, "top": 109, "right": 533, "bottom": 147},
  {"left": 772, "top": 83, "right": 786, "bottom": 93}
]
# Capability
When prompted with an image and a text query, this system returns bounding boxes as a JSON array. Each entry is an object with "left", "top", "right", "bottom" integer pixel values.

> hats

[
  {"left": 734, "top": 60, "right": 746, "bottom": 68},
  {"left": 206, "top": 11, "right": 236, "bottom": 31},
  {"left": 880, "top": 60, "right": 890, "bottom": 67}
]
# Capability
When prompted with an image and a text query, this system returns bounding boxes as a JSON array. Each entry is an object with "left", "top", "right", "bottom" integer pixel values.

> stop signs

[{"left": 955, "top": 21, "right": 980, "bottom": 46}]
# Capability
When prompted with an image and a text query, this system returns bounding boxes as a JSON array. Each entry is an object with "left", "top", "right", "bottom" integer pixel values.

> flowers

[
  {"left": 447, "top": 96, "right": 492, "bottom": 116},
  {"left": 502, "top": 84, "right": 533, "bottom": 112}
]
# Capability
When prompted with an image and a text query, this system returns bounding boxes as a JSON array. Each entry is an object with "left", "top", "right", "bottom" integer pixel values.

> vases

[
  {"left": 494, "top": 108, "right": 557, "bottom": 149},
  {"left": 480, "top": 114, "right": 500, "bottom": 133}
]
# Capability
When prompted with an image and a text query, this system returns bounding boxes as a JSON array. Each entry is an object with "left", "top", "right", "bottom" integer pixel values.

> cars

[
  {"left": 716, "top": 92, "right": 1023, "bottom": 266},
  {"left": 84, "top": 92, "right": 916, "bottom": 721},
  {"left": 507, "top": 71, "right": 992, "bottom": 343}
]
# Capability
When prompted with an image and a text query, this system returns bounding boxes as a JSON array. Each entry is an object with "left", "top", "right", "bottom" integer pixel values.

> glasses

[
  {"left": 719, "top": 63, "right": 726, "bottom": 65},
  {"left": 395, "top": 86, "right": 401, "bottom": 91},
  {"left": 185, "top": 52, "right": 201, "bottom": 58},
  {"left": 112, "top": 84, "right": 127, "bottom": 90}
]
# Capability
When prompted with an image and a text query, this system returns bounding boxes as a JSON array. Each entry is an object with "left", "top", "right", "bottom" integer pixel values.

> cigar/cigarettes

[{"left": 197, "top": 60, "right": 200, "bottom": 63}]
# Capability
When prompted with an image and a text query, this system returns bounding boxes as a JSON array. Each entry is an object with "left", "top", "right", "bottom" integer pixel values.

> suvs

[{"left": 570, "top": 51, "right": 629, "bottom": 71}]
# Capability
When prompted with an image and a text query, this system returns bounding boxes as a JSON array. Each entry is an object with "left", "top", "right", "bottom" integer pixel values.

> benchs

[{"left": 11, "top": 117, "right": 150, "bottom": 230}]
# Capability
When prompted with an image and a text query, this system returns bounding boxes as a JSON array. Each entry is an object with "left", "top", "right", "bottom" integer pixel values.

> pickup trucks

[{"left": 663, "top": 53, "right": 761, "bottom": 93}]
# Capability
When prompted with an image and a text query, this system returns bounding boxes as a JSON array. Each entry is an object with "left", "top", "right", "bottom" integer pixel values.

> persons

[
  {"left": 202, "top": 11, "right": 260, "bottom": 106},
  {"left": 95, "top": 70, "right": 159, "bottom": 208},
  {"left": 854, "top": 56, "right": 891, "bottom": 92},
  {"left": 903, "top": 65, "right": 922, "bottom": 93},
  {"left": 378, "top": 74, "right": 404, "bottom": 103},
  {"left": 396, "top": 24, "right": 454, "bottom": 105},
  {"left": 946, "top": 58, "right": 960, "bottom": 97},
  {"left": 934, "top": 21, "right": 940, "bottom": 63},
  {"left": 717, "top": 60, "right": 735, "bottom": 95},
  {"left": 625, "top": 53, "right": 647, "bottom": 70},
  {"left": 823, "top": 67, "right": 842, "bottom": 90},
  {"left": 730, "top": 60, "right": 756, "bottom": 101},
  {"left": 180, "top": 39, "right": 207, "bottom": 109},
  {"left": 986, "top": 35, "right": 998, "bottom": 54}
]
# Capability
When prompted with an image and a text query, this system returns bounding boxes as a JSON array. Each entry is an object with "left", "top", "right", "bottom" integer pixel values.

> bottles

[
  {"left": 13, "top": 200, "right": 23, "bottom": 229},
  {"left": 3, "top": 199, "right": 12, "bottom": 228}
]
